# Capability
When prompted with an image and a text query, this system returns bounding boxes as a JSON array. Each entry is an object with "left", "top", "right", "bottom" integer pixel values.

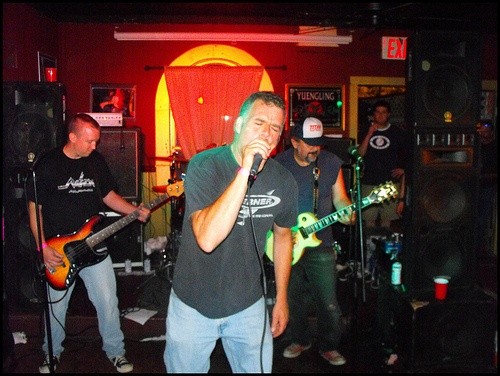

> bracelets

[
  {"left": 237, "top": 167, "right": 256, "bottom": 179},
  {"left": 36, "top": 242, "right": 48, "bottom": 251}
]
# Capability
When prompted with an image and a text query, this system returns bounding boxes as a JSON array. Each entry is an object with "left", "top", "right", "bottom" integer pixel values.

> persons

[
  {"left": 273, "top": 116, "right": 356, "bottom": 365},
  {"left": 354, "top": 100, "right": 407, "bottom": 228},
  {"left": 26, "top": 114, "right": 151, "bottom": 374},
  {"left": 163, "top": 90, "right": 299, "bottom": 375}
]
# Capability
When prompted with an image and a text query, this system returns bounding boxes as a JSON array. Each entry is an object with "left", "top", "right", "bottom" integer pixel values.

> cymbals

[
  {"left": 150, "top": 156, "right": 188, "bottom": 162},
  {"left": 151, "top": 185, "right": 168, "bottom": 193}
]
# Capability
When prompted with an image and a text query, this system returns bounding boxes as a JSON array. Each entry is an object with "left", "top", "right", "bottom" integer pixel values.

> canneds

[
  {"left": 144, "top": 259, "right": 150, "bottom": 273},
  {"left": 125, "top": 260, "right": 131, "bottom": 272},
  {"left": 392, "top": 262, "right": 402, "bottom": 285}
]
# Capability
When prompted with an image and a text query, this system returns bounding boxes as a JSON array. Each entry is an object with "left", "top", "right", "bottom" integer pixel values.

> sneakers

[
  {"left": 109, "top": 355, "right": 134, "bottom": 373},
  {"left": 282, "top": 342, "right": 312, "bottom": 358},
  {"left": 39, "top": 354, "right": 56, "bottom": 373},
  {"left": 321, "top": 350, "right": 346, "bottom": 365}
]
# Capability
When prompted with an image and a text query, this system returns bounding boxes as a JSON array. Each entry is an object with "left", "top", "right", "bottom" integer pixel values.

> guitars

[
  {"left": 38, "top": 173, "right": 184, "bottom": 291},
  {"left": 264, "top": 180, "right": 399, "bottom": 265}
]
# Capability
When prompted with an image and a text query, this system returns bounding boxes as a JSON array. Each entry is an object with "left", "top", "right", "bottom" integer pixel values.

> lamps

[{"left": 113, "top": 26, "right": 356, "bottom": 45}]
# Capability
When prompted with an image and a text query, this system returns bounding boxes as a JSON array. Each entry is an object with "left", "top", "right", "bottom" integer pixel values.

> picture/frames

[
  {"left": 284, "top": 83, "right": 347, "bottom": 133},
  {"left": 37, "top": 51, "right": 58, "bottom": 82},
  {"left": 90, "top": 83, "right": 137, "bottom": 120}
]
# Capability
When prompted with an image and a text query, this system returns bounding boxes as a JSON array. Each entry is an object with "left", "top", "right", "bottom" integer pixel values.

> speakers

[
  {"left": 3, "top": 80, "right": 67, "bottom": 174},
  {"left": 99, "top": 211, "right": 144, "bottom": 268},
  {"left": 411, "top": 145, "right": 481, "bottom": 292},
  {"left": 376, "top": 273, "right": 498, "bottom": 376},
  {"left": 414, "top": 28, "right": 481, "bottom": 128},
  {"left": 3, "top": 178, "right": 48, "bottom": 314},
  {"left": 96, "top": 130, "right": 140, "bottom": 202}
]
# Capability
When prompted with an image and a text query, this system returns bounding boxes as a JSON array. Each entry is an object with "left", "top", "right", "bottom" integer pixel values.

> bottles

[
  {"left": 143, "top": 257, "right": 151, "bottom": 273},
  {"left": 390, "top": 259, "right": 403, "bottom": 285},
  {"left": 335, "top": 240, "right": 339, "bottom": 255},
  {"left": 125, "top": 259, "right": 133, "bottom": 273}
]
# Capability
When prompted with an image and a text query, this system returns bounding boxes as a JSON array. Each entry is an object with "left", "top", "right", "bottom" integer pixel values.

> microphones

[
  {"left": 348, "top": 145, "right": 364, "bottom": 163},
  {"left": 249, "top": 153, "right": 263, "bottom": 183},
  {"left": 120, "top": 132, "right": 124, "bottom": 152}
]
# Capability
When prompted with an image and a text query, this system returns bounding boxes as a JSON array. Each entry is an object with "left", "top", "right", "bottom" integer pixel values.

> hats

[{"left": 291, "top": 117, "right": 329, "bottom": 146}]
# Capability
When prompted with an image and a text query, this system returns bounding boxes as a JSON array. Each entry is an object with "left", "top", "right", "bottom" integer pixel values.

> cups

[{"left": 434, "top": 278, "right": 449, "bottom": 300}]
